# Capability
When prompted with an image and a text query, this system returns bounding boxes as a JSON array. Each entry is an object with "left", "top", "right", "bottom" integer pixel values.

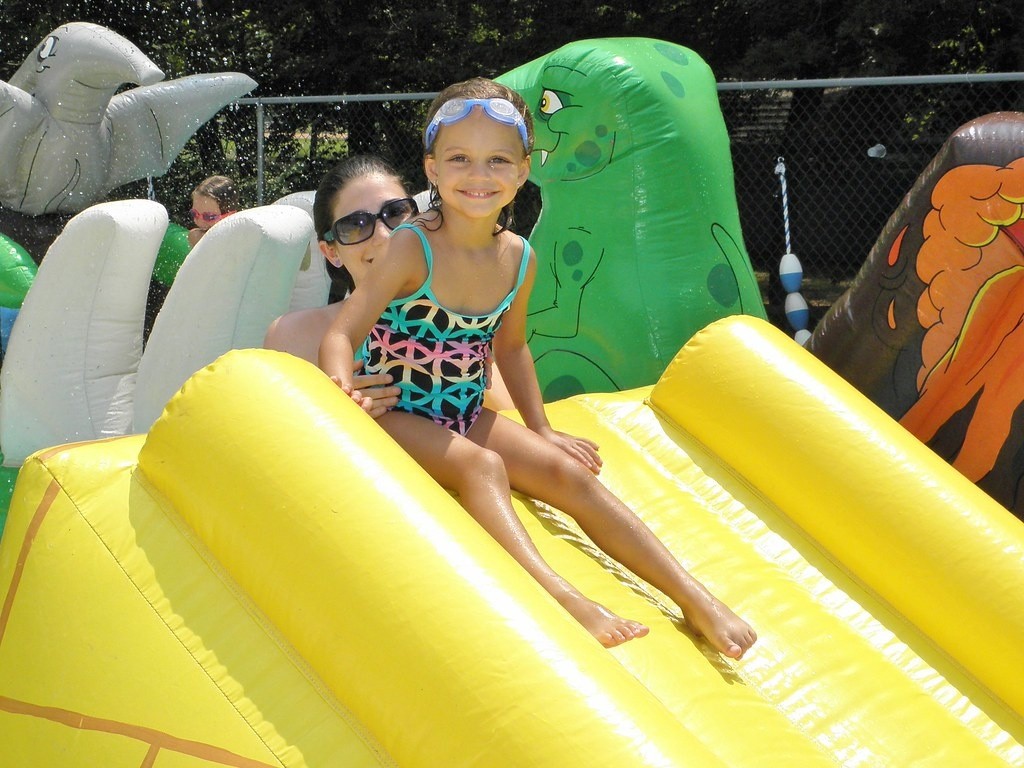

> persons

[
  {"left": 189, "top": 176, "right": 240, "bottom": 248},
  {"left": 318, "top": 77, "right": 758, "bottom": 660},
  {"left": 262, "top": 154, "right": 516, "bottom": 418}
]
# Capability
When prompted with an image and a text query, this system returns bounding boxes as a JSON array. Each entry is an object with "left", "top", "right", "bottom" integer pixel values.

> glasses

[
  {"left": 424, "top": 98, "right": 529, "bottom": 157},
  {"left": 321, "top": 197, "right": 420, "bottom": 247},
  {"left": 189, "top": 209, "right": 237, "bottom": 222}
]
